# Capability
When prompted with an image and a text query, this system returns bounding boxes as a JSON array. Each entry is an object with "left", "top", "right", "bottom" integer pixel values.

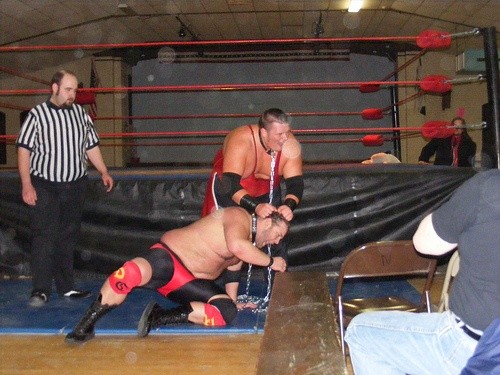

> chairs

[{"left": 334, "top": 241, "right": 437, "bottom": 366}]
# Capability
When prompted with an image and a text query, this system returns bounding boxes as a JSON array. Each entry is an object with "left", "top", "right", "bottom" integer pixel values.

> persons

[
  {"left": 417, "top": 117, "right": 477, "bottom": 168},
  {"left": 200, "top": 108, "right": 304, "bottom": 280},
  {"left": 16, "top": 69, "right": 113, "bottom": 307},
  {"left": 344, "top": 169, "right": 500, "bottom": 375},
  {"left": 63, "top": 206, "right": 290, "bottom": 347}
]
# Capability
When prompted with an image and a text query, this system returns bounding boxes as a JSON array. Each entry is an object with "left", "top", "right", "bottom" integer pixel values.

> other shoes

[
  {"left": 61, "top": 289, "right": 91, "bottom": 298},
  {"left": 30, "top": 291, "right": 49, "bottom": 306}
]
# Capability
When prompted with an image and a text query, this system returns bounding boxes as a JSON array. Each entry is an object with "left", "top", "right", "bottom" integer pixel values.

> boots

[
  {"left": 138, "top": 301, "right": 196, "bottom": 337},
  {"left": 64, "top": 293, "right": 116, "bottom": 343}
]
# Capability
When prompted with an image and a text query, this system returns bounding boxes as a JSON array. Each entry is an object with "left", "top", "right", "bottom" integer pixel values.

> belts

[{"left": 455, "top": 318, "right": 481, "bottom": 341}]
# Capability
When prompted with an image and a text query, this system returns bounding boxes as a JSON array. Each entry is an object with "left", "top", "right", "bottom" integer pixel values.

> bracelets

[{"left": 266, "top": 256, "right": 274, "bottom": 267}]
